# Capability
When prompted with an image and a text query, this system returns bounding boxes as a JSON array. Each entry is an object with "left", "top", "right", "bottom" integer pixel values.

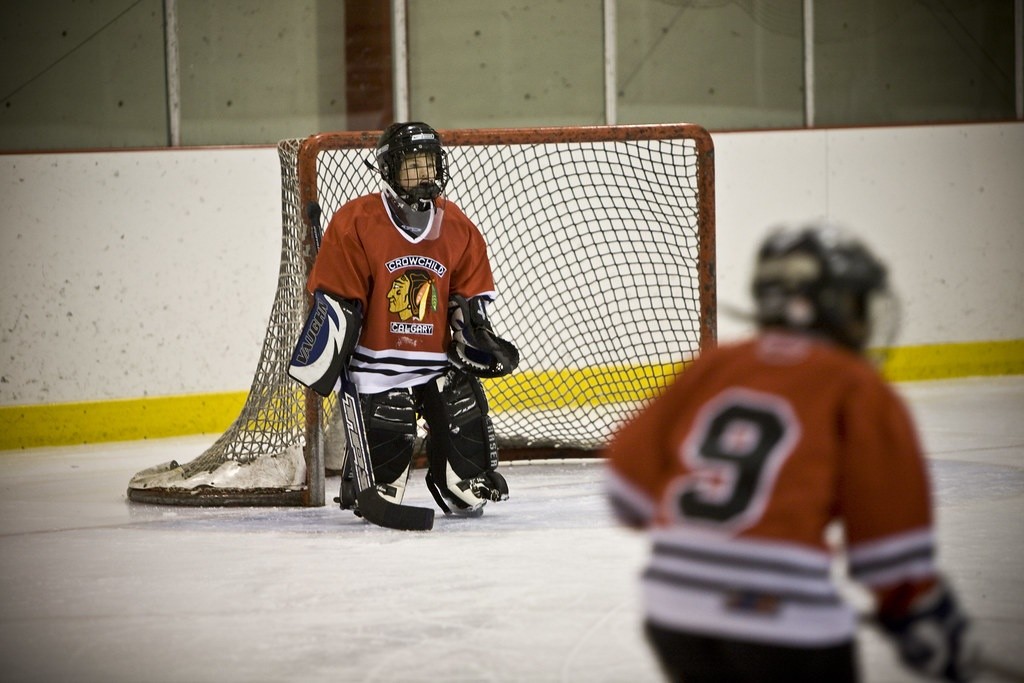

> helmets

[
  {"left": 376, "top": 121, "right": 449, "bottom": 203},
  {"left": 753, "top": 222, "right": 902, "bottom": 367}
]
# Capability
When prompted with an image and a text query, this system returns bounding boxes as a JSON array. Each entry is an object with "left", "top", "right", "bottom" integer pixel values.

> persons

[
  {"left": 606, "top": 225, "right": 1024, "bottom": 683},
  {"left": 287, "top": 121, "right": 519, "bottom": 531}
]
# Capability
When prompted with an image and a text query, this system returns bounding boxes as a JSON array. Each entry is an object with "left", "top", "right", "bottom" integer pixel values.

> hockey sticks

[{"left": 308, "top": 200, "right": 435, "bottom": 532}]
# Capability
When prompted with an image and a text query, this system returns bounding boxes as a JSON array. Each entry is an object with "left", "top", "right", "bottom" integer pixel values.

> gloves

[{"left": 874, "top": 576, "right": 981, "bottom": 683}]
